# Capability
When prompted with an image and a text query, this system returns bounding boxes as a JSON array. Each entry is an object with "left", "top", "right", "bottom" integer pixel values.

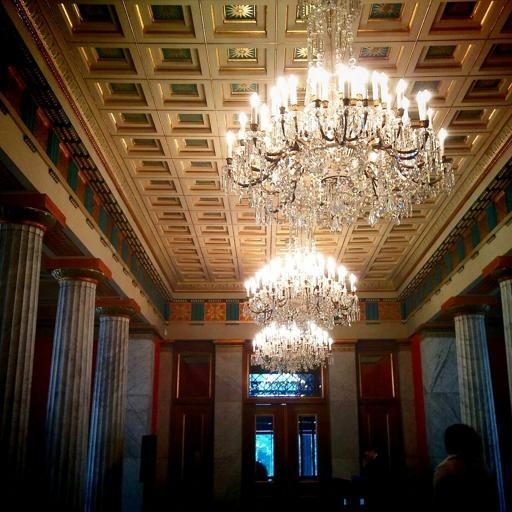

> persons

[
  {"left": 430, "top": 424, "right": 497, "bottom": 511},
  {"left": 344, "top": 435, "right": 386, "bottom": 491}
]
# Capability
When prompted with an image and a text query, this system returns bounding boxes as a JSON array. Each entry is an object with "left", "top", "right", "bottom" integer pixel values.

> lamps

[
  {"left": 248, "top": 320, "right": 335, "bottom": 373},
  {"left": 243, "top": 227, "right": 361, "bottom": 329},
  {"left": 258, "top": 375, "right": 308, "bottom": 396},
  {"left": 220, "top": 0, "right": 456, "bottom": 232}
]
